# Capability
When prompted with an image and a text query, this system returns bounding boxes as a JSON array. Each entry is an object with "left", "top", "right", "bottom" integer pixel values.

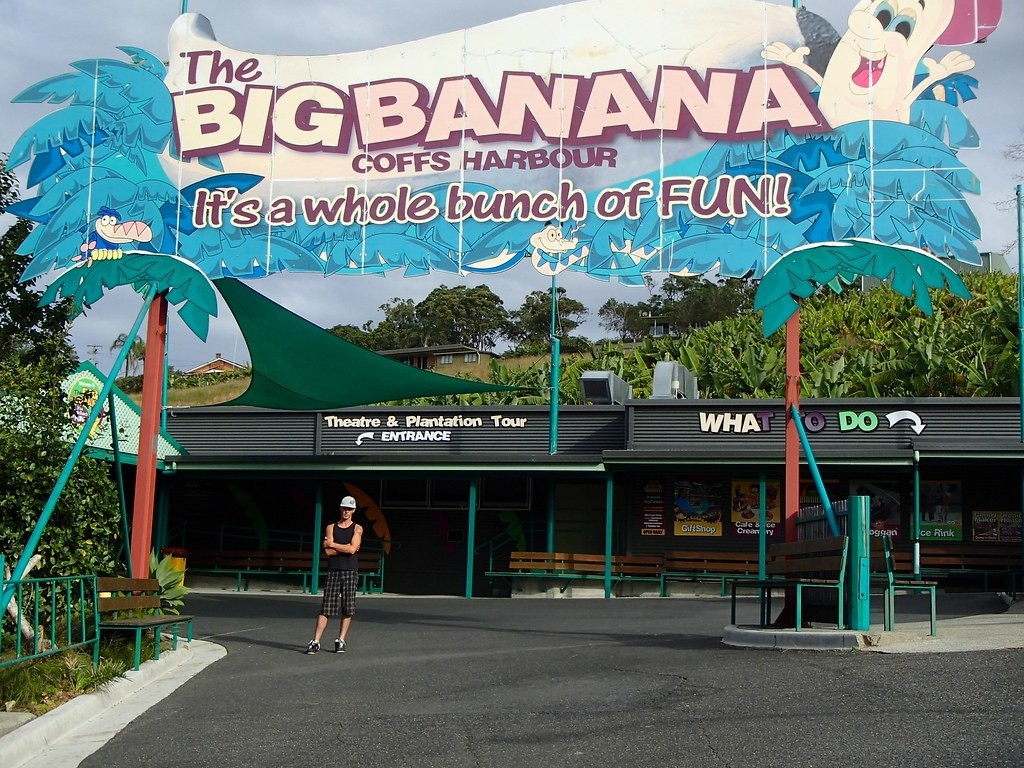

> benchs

[
  {"left": 729, "top": 534, "right": 850, "bottom": 632},
  {"left": 870, "top": 533, "right": 939, "bottom": 637},
  {"left": 92, "top": 577, "right": 195, "bottom": 671},
  {"left": 485, "top": 551, "right": 664, "bottom": 600},
  {"left": 180, "top": 546, "right": 385, "bottom": 596},
  {"left": 661, "top": 547, "right": 914, "bottom": 597},
  {"left": 913, "top": 540, "right": 1024, "bottom": 592}
]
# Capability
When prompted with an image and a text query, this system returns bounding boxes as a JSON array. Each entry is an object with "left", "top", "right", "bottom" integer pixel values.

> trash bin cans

[{"left": 162, "top": 547, "right": 189, "bottom": 590}]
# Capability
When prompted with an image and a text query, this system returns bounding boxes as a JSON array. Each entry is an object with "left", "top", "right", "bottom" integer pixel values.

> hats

[{"left": 339, "top": 496, "right": 356, "bottom": 510}]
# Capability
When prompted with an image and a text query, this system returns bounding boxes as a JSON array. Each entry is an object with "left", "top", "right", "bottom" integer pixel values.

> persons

[{"left": 305, "top": 495, "right": 364, "bottom": 656}]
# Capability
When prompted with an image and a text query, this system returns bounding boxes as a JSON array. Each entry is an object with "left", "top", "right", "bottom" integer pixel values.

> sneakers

[
  {"left": 307, "top": 640, "right": 321, "bottom": 656},
  {"left": 334, "top": 638, "right": 346, "bottom": 655}
]
